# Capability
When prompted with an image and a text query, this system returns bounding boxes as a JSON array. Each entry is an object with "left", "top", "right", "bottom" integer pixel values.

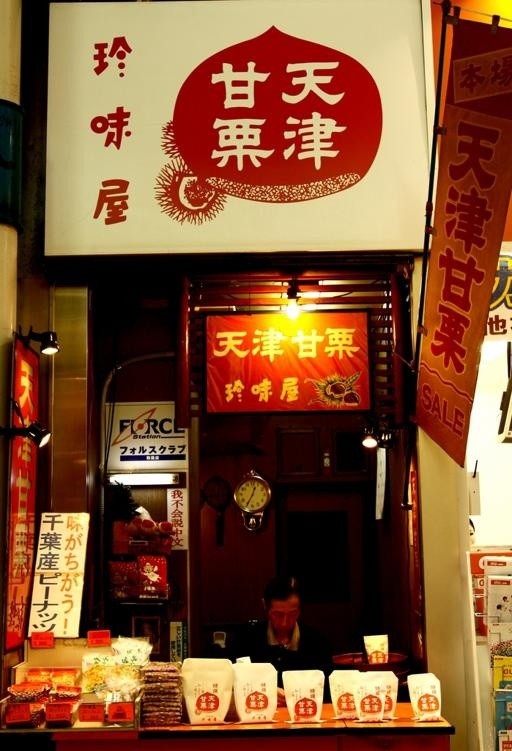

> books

[{"left": 470, "top": 552, "right": 512, "bottom": 751}]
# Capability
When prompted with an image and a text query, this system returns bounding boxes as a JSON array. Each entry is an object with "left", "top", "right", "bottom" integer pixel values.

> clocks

[{"left": 234, "top": 470, "right": 274, "bottom": 532}]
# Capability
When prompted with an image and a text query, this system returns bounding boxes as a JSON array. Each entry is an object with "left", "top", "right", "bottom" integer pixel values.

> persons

[{"left": 238, "top": 572, "right": 333, "bottom": 708}]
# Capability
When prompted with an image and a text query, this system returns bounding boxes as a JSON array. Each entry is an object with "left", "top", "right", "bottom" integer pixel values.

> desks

[
  {"left": 50, "top": 701, "right": 346, "bottom": 751},
  {"left": 340, "top": 697, "right": 456, "bottom": 750}
]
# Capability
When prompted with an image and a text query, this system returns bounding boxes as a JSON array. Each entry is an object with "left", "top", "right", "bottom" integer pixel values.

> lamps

[
  {"left": 0, "top": 398, "right": 54, "bottom": 452},
  {"left": 358, "top": 424, "right": 398, "bottom": 451},
  {"left": 282, "top": 282, "right": 302, "bottom": 321},
  {"left": 106, "top": 471, "right": 181, "bottom": 488},
  {"left": 22, "top": 325, "right": 62, "bottom": 359}
]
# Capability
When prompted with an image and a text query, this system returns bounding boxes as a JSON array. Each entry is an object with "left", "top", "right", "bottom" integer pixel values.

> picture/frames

[{"left": 129, "top": 613, "right": 163, "bottom": 657}]
